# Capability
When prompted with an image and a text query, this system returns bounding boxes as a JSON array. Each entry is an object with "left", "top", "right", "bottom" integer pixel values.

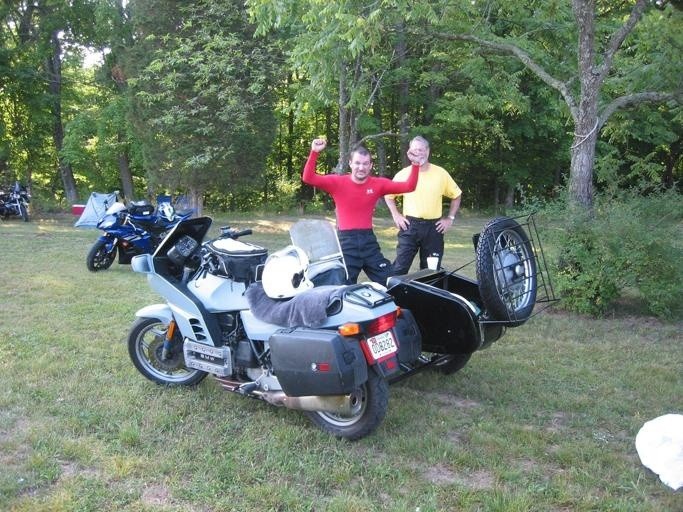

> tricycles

[{"left": 127, "top": 210, "right": 560, "bottom": 442}]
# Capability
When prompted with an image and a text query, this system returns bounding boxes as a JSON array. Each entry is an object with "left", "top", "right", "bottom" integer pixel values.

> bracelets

[{"left": 446, "top": 215, "right": 455, "bottom": 220}]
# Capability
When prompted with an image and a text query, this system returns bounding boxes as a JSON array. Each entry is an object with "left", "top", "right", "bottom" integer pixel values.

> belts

[
  {"left": 406, "top": 215, "right": 440, "bottom": 225},
  {"left": 337, "top": 229, "right": 373, "bottom": 236}
]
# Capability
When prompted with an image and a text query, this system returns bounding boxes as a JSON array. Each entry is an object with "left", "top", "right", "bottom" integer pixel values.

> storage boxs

[{"left": 72, "top": 205, "right": 85, "bottom": 215}]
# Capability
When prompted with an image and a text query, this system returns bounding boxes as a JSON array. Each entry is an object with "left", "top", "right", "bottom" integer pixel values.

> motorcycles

[
  {"left": 86, "top": 193, "right": 193, "bottom": 272},
  {"left": 0, "top": 180, "right": 31, "bottom": 222}
]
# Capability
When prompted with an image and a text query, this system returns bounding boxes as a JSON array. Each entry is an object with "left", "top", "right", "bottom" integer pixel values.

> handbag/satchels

[{"left": 202, "top": 237, "right": 268, "bottom": 296}]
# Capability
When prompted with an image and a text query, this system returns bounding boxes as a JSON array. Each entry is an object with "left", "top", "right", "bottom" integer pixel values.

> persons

[
  {"left": 382, "top": 135, "right": 462, "bottom": 275},
  {"left": 301, "top": 137, "right": 426, "bottom": 286}
]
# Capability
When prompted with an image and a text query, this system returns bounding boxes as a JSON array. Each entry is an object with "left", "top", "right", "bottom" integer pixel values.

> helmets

[
  {"left": 261, "top": 245, "right": 314, "bottom": 299},
  {"left": 159, "top": 202, "right": 175, "bottom": 222}
]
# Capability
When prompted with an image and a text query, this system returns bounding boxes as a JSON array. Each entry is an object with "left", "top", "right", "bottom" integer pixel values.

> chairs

[{"left": 290, "top": 220, "right": 349, "bottom": 285}]
